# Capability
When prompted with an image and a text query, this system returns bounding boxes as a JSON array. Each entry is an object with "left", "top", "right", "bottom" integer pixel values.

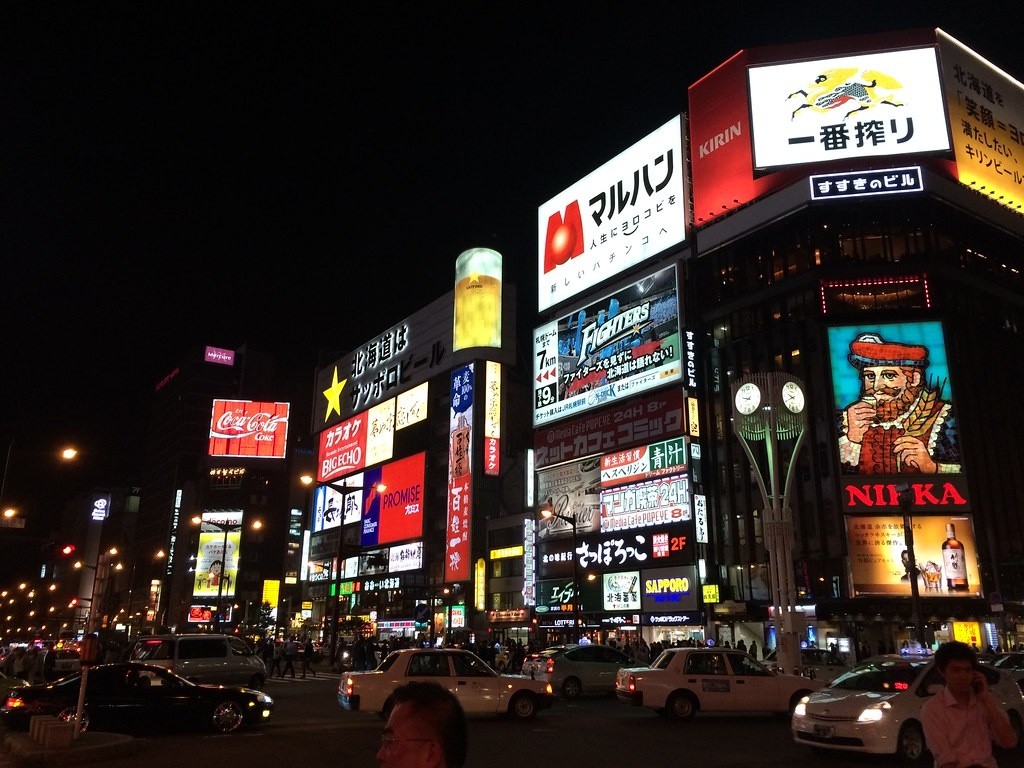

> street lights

[
  {"left": 0, "top": 441, "right": 78, "bottom": 507},
  {"left": 191, "top": 517, "right": 263, "bottom": 631},
  {"left": 298, "top": 475, "right": 387, "bottom": 664},
  {"left": 541, "top": 510, "right": 579, "bottom": 644},
  {"left": 116, "top": 550, "right": 165, "bottom": 640}
]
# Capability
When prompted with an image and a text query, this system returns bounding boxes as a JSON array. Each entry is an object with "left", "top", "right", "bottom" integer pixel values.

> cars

[
  {"left": 487, "top": 643, "right": 520, "bottom": 672},
  {"left": 279, "top": 640, "right": 305, "bottom": 661},
  {"left": 299, "top": 635, "right": 389, "bottom": 659},
  {"left": 337, "top": 648, "right": 554, "bottom": 722},
  {"left": 792, "top": 653, "right": 1023, "bottom": 766},
  {"left": 1, "top": 660, "right": 275, "bottom": 734},
  {"left": 758, "top": 647, "right": 1023, "bottom": 691},
  {"left": 521, "top": 643, "right": 650, "bottom": 704},
  {"left": 1, "top": 647, "right": 81, "bottom": 706},
  {"left": 614, "top": 646, "right": 823, "bottom": 723}
]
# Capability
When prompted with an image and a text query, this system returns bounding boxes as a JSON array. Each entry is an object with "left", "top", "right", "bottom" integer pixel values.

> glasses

[{"left": 380, "top": 734, "right": 440, "bottom": 751}]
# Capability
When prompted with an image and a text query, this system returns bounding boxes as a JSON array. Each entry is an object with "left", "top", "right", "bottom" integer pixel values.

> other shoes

[{"left": 268, "top": 671, "right": 318, "bottom": 679}]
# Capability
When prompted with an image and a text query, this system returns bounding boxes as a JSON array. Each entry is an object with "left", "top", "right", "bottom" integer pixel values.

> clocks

[
  {"left": 782, "top": 381, "right": 805, "bottom": 414},
  {"left": 734, "top": 382, "right": 761, "bottom": 416}
]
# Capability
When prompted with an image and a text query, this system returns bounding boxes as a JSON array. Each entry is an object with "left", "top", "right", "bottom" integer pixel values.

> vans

[{"left": 129, "top": 633, "right": 268, "bottom": 692}]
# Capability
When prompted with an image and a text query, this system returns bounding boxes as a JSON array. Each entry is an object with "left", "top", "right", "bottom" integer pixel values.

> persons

[
  {"left": 921, "top": 641, "right": 1017, "bottom": 768},
  {"left": 985, "top": 644, "right": 1023, "bottom": 654},
  {"left": 809, "top": 641, "right": 840, "bottom": 659},
  {"left": 713, "top": 640, "right": 772, "bottom": 662},
  {"left": 377, "top": 680, "right": 470, "bottom": 768},
  {"left": 331, "top": 628, "right": 559, "bottom": 678},
  {"left": 240, "top": 638, "right": 282, "bottom": 678},
  {"left": 280, "top": 636, "right": 297, "bottom": 678},
  {"left": 299, "top": 638, "right": 316, "bottom": 679},
  {"left": 862, "top": 639, "right": 896, "bottom": 658},
  {"left": 138, "top": 675, "right": 165, "bottom": 689},
  {"left": 0, "top": 638, "right": 132, "bottom": 683},
  {"left": 610, "top": 637, "right": 709, "bottom": 665}
]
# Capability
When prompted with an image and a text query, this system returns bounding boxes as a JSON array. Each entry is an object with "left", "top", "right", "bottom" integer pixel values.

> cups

[{"left": 919, "top": 563, "right": 942, "bottom": 589}]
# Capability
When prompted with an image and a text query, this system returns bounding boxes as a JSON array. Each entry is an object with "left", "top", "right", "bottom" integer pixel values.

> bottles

[{"left": 941, "top": 524, "right": 969, "bottom": 591}]
